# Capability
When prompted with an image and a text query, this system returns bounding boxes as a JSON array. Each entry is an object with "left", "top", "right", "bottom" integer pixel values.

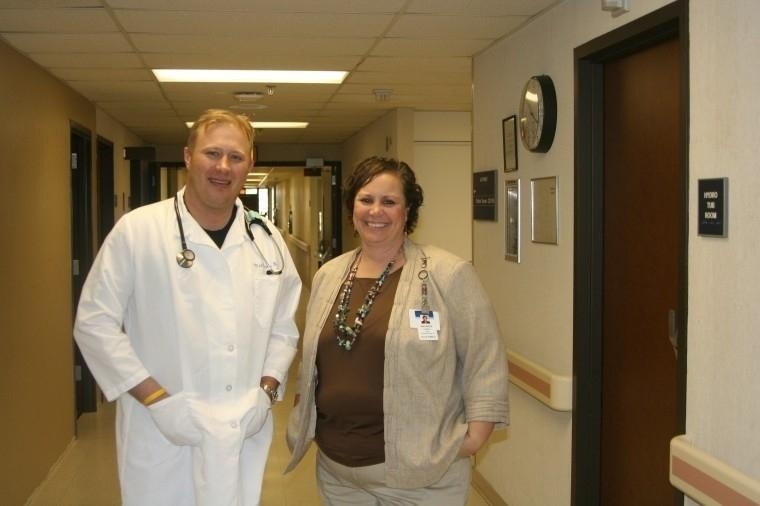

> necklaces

[{"left": 335, "top": 250, "right": 397, "bottom": 351}]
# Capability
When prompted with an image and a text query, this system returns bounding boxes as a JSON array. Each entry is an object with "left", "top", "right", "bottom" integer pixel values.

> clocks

[{"left": 518, "top": 76, "right": 557, "bottom": 154}]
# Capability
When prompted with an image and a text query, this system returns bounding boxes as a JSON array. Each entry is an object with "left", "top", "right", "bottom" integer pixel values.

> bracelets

[{"left": 142, "top": 388, "right": 166, "bottom": 408}]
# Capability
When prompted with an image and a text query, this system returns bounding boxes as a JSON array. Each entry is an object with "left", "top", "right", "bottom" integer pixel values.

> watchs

[{"left": 258, "top": 380, "right": 279, "bottom": 404}]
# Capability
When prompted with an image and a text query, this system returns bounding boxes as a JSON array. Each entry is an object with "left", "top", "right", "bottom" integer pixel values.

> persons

[
  {"left": 282, "top": 154, "right": 509, "bottom": 506},
  {"left": 71, "top": 110, "right": 302, "bottom": 505}
]
